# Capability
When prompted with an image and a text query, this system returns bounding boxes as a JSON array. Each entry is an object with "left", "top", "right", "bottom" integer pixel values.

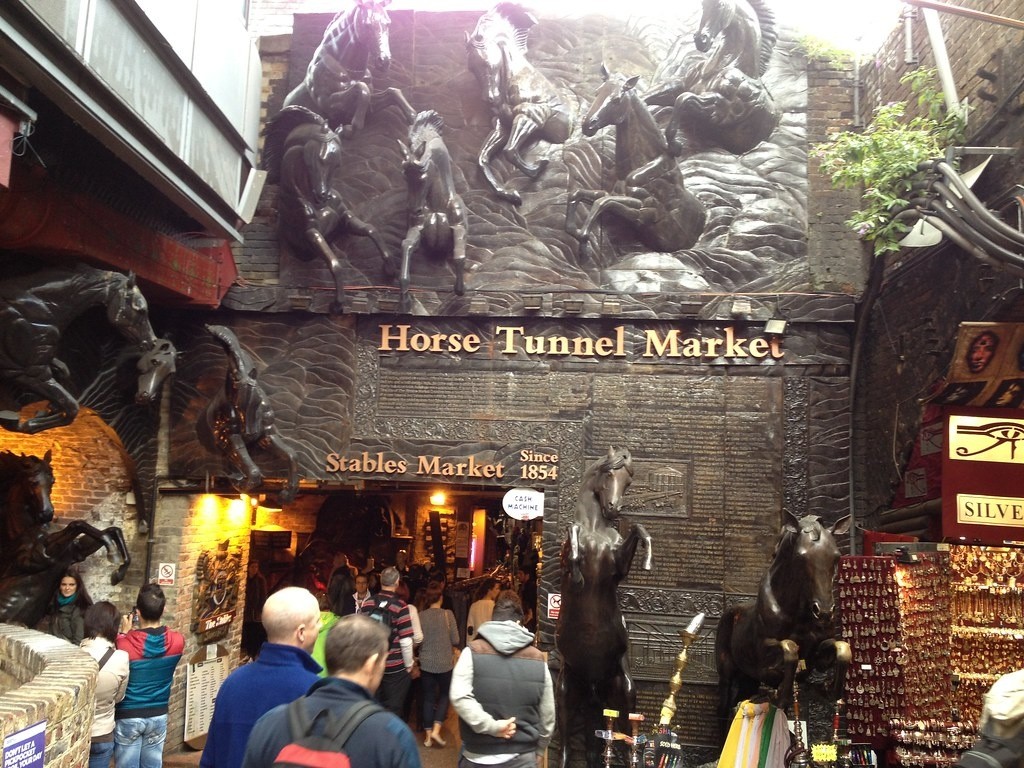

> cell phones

[{"left": 132, "top": 606, "right": 140, "bottom": 622}]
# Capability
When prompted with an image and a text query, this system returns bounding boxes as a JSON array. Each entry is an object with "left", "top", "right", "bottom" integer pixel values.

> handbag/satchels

[{"left": 454, "top": 650, "right": 461, "bottom": 665}]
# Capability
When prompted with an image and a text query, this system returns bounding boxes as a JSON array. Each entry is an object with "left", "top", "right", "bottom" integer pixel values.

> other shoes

[
  {"left": 425, "top": 739, "right": 433, "bottom": 747},
  {"left": 431, "top": 734, "right": 448, "bottom": 746}
]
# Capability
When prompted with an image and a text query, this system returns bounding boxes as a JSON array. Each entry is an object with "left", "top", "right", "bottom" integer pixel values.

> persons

[
  {"left": 241, "top": 559, "right": 267, "bottom": 662},
  {"left": 49, "top": 568, "right": 94, "bottom": 646},
  {"left": 957, "top": 667, "right": 1024, "bottom": 768},
  {"left": 199, "top": 586, "right": 323, "bottom": 768},
  {"left": 449, "top": 601, "right": 556, "bottom": 768},
  {"left": 240, "top": 612, "right": 424, "bottom": 768},
  {"left": 114, "top": 584, "right": 185, "bottom": 768},
  {"left": 330, "top": 550, "right": 538, "bottom": 747},
  {"left": 78, "top": 602, "right": 129, "bottom": 768}
]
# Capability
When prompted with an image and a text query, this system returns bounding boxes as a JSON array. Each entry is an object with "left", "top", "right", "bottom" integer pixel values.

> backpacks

[
  {"left": 274, "top": 695, "right": 385, "bottom": 767},
  {"left": 959, "top": 717, "right": 1023, "bottom": 768},
  {"left": 368, "top": 595, "right": 401, "bottom": 651}
]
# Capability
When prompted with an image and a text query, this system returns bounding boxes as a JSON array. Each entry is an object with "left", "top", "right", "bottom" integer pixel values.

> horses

[
  {"left": 260, "top": 105, "right": 396, "bottom": 313},
  {"left": 280, "top": 0, "right": 417, "bottom": 139},
  {"left": 713, "top": 508, "right": 854, "bottom": 751},
  {"left": 1, "top": 261, "right": 159, "bottom": 434},
  {"left": 640, "top": 0, "right": 777, "bottom": 157},
  {"left": 464, "top": 1, "right": 575, "bottom": 205},
  {"left": 555, "top": 443, "right": 652, "bottom": 754},
  {"left": 196, "top": 324, "right": 301, "bottom": 504},
  {"left": 396, "top": 109, "right": 470, "bottom": 314},
  {"left": 196, "top": 549, "right": 218, "bottom": 619},
  {"left": 1, "top": 447, "right": 132, "bottom": 587},
  {"left": 221, "top": 545, "right": 243, "bottom": 612},
  {"left": 115, "top": 339, "right": 177, "bottom": 403},
  {"left": 563, "top": 63, "right": 708, "bottom": 265}
]
